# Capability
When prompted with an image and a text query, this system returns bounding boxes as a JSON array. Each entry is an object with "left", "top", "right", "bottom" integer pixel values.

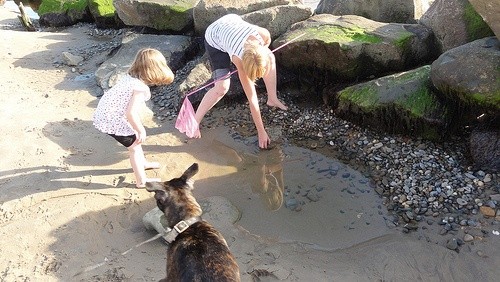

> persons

[
  {"left": 188, "top": 12, "right": 288, "bottom": 150},
  {"left": 93, "top": 48, "right": 176, "bottom": 188}
]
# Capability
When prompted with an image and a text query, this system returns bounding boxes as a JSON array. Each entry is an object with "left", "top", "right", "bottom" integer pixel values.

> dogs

[{"left": 145, "top": 163, "right": 241, "bottom": 282}]
